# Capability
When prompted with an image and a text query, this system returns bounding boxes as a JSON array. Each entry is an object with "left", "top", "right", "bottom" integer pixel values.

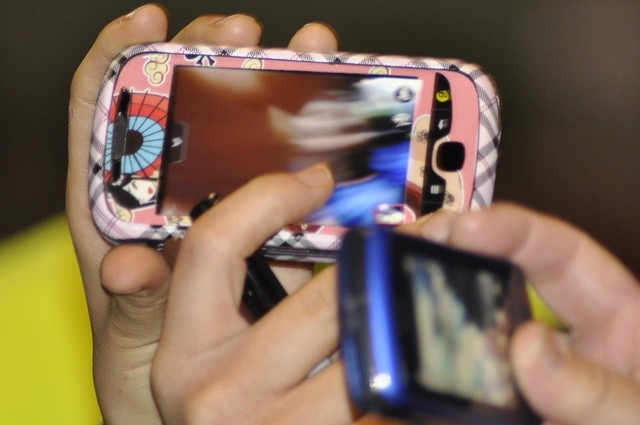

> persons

[
  {"left": 148, "top": 163, "right": 640, "bottom": 425},
  {"left": 61, "top": 2, "right": 340, "bottom": 425}
]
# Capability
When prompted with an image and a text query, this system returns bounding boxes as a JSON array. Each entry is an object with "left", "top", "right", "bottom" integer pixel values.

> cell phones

[
  {"left": 86, "top": 42, "right": 502, "bottom": 264},
  {"left": 337, "top": 226, "right": 533, "bottom": 425}
]
